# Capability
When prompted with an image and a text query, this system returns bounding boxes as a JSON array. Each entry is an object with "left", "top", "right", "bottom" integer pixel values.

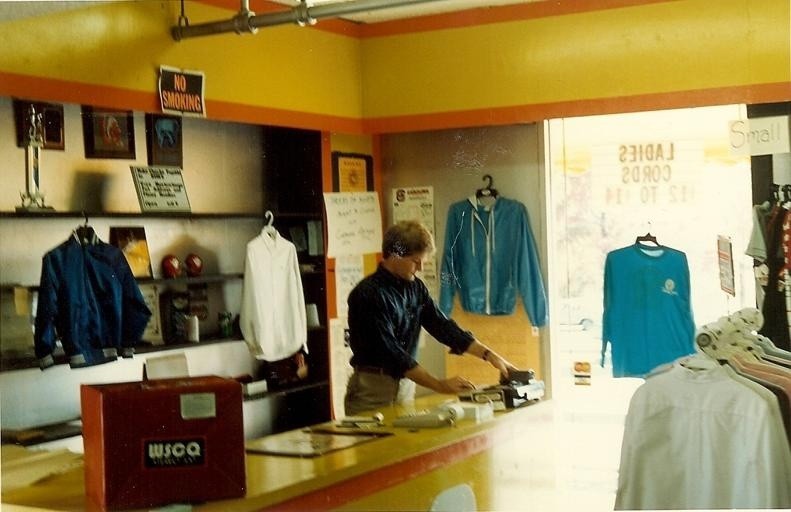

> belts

[{"left": 357, "top": 365, "right": 391, "bottom": 375}]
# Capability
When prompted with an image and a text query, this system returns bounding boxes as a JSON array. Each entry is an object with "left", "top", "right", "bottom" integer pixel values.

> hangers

[
  {"left": 635, "top": 221, "right": 661, "bottom": 253},
  {"left": 767, "top": 183, "right": 791, "bottom": 205},
  {"left": 69, "top": 208, "right": 101, "bottom": 246},
  {"left": 679, "top": 307, "right": 772, "bottom": 376},
  {"left": 259, "top": 210, "right": 279, "bottom": 241},
  {"left": 475, "top": 175, "right": 498, "bottom": 208}
]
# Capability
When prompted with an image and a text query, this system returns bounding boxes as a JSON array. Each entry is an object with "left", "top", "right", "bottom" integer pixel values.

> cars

[{"left": 557, "top": 296, "right": 604, "bottom": 333}]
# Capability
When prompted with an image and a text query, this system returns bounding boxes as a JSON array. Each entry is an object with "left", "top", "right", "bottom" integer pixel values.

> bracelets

[{"left": 482, "top": 349, "right": 491, "bottom": 360}]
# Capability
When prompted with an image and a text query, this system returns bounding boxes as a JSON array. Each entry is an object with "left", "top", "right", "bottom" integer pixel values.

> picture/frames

[
  {"left": 145, "top": 113, "right": 183, "bottom": 168},
  {"left": 12, "top": 100, "right": 65, "bottom": 150},
  {"left": 81, "top": 105, "right": 137, "bottom": 160}
]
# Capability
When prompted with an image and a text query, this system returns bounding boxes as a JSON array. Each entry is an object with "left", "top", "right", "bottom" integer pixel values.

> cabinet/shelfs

[{"left": 0, "top": 208, "right": 329, "bottom": 451}]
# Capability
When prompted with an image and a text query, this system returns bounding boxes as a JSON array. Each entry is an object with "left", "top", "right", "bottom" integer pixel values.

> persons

[
  {"left": 26, "top": 103, "right": 37, "bottom": 139},
  {"left": 344, "top": 219, "right": 522, "bottom": 418},
  {"left": 35, "top": 110, "right": 43, "bottom": 136}
]
